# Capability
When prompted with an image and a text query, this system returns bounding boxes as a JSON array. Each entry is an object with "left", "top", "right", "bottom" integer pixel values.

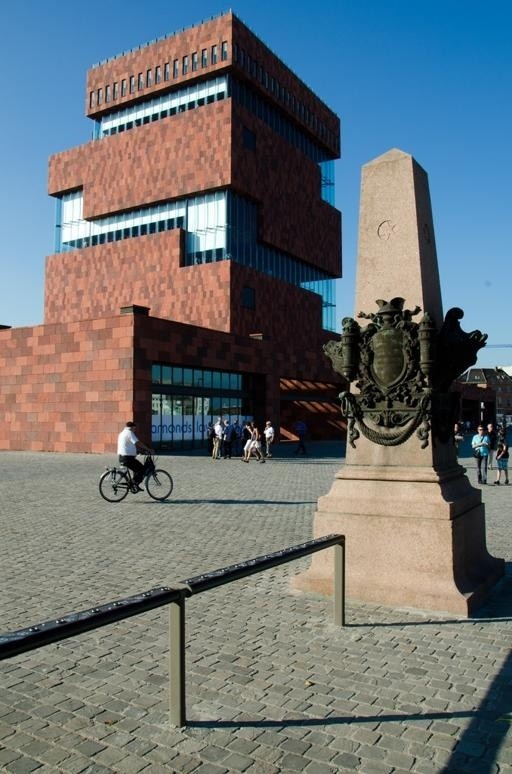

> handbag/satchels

[
  {"left": 472, "top": 448, "right": 480, "bottom": 457},
  {"left": 144, "top": 456, "right": 155, "bottom": 475}
]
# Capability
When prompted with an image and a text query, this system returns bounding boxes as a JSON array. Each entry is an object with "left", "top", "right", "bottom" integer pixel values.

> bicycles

[{"left": 96, "top": 447, "right": 173, "bottom": 501}]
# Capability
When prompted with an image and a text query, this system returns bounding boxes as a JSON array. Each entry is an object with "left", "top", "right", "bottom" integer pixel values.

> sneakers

[
  {"left": 494, "top": 480, "right": 500, "bottom": 484},
  {"left": 504, "top": 480, "right": 509, "bottom": 484}
]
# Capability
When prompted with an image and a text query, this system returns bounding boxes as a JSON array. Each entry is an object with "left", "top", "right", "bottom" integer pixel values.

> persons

[
  {"left": 471, "top": 424, "right": 492, "bottom": 484},
  {"left": 486, "top": 422, "right": 496, "bottom": 471},
  {"left": 293, "top": 418, "right": 308, "bottom": 454},
  {"left": 206, "top": 412, "right": 281, "bottom": 463},
  {"left": 116, "top": 420, "right": 155, "bottom": 492},
  {"left": 453, "top": 423, "right": 463, "bottom": 463},
  {"left": 493, "top": 429, "right": 510, "bottom": 485}
]
